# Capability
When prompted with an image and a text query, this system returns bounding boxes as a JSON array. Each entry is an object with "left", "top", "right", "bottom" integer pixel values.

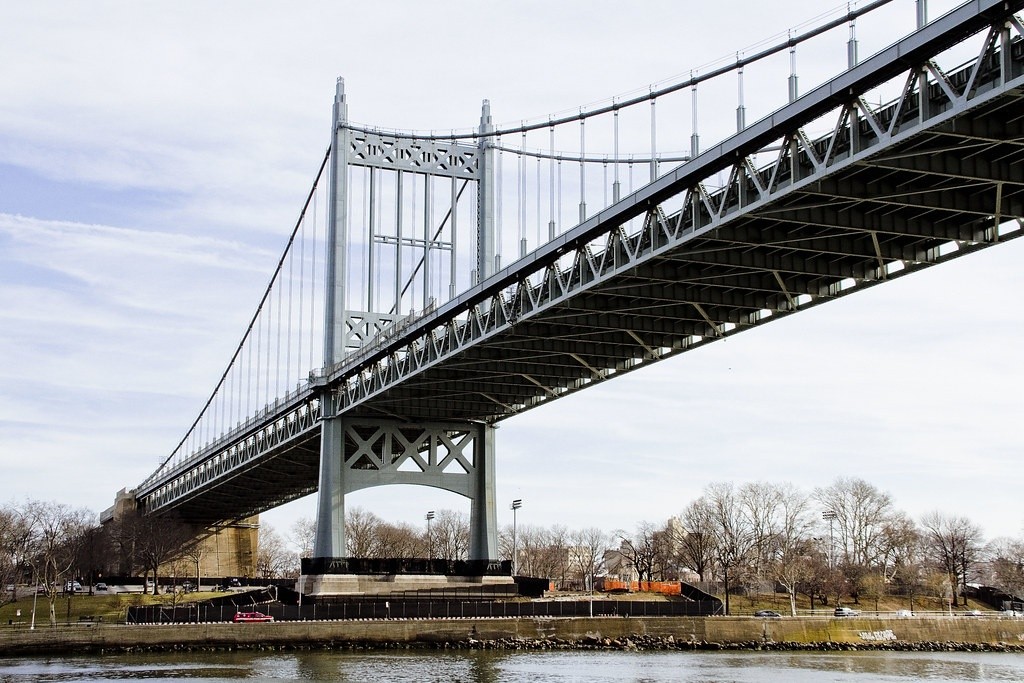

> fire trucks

[{"left": 234, "top": 612, "right": 274, "bottom": 622}]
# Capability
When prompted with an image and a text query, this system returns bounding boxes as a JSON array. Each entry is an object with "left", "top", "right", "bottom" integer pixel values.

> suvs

[{"left": 834, "top": 608, "right": 859, "bottom": 617}]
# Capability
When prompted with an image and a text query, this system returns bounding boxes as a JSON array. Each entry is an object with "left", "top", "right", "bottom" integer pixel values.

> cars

[
  {"left": 65, "top": 581, "right": 83, "bottom": 591},
  {"left": 96, "top": 583, "right": 109, "bottom": 591},
  {"left": 965, "top": 610, "right": 982, "bottom": 616},
  {"left": 755, "top": 610, "right": 781, "bottom": 617},
  {"left": 898, "top": 610, "right": 916, "bottom": 617},
  {"left": 166, "top": 586, "right": 178, "bottom": 593}
]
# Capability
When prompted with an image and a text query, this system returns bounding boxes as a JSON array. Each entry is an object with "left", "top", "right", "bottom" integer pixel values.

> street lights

[{"left": 31, "top": 559, "right": 39, "bottom": 629}]
